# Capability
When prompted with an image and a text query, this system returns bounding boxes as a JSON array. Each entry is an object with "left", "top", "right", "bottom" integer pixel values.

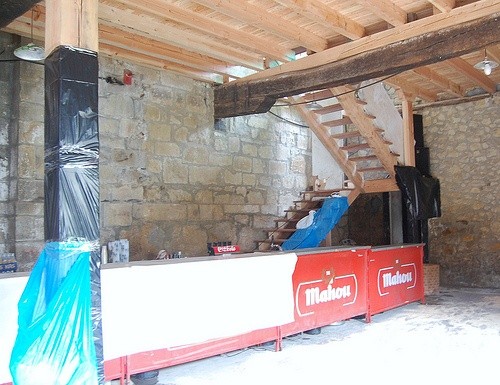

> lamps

[
  {"left": 473, "top": 46, "right": 498, "bottom": 76},
  {"left": 13, "top": 9, "right": 47, "bottom": 61}
]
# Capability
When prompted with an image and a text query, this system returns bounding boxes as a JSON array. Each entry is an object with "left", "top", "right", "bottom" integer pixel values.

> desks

[{"left": 0, "top": 242, "right": 426, "bottom": 385}]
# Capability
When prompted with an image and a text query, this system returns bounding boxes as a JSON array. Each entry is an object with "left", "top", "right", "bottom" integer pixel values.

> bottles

[
  {"left": 0, "top": 253, "right": 17, "bottom": 273},
  {"left": 207, "top": 241, "right": 232, "bottom": 256},
  {"left": 270, "top": 236, "right": 275, "bottom": 251},
  {"left": 314, "top": 176, "right": 321, "bottom": 191},
  {"left": 166, "top": 251, "right": 182, "bottom": 259}
]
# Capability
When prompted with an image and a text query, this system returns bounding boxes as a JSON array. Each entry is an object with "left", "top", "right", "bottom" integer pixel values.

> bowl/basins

[{"left": 131, "top": 370, "right": 159, "bottom": 385}]
